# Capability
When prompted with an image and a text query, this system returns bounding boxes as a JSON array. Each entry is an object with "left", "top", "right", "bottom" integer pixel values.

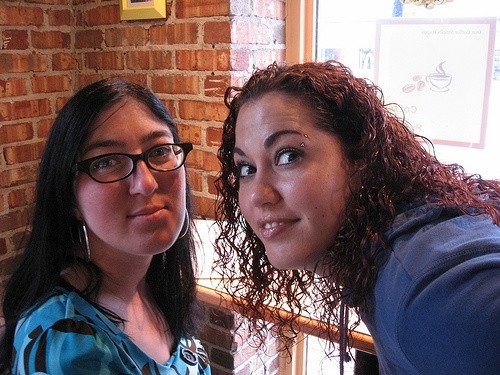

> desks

[{"left": 194, "top": 216, "right": 376, "bottom": 358}]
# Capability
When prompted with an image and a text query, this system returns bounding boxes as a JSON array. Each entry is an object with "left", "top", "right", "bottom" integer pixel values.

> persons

[
  {"left": 1, "top": 76, "right": 210, "bottom": 375},
  {"left": 210, "top": 59, "right": 499, "bottom": 375}
]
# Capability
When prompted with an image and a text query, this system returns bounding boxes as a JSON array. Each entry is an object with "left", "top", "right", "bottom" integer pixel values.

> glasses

[{"left": 72, "top": 142, "right": 193, "bottom": 183}]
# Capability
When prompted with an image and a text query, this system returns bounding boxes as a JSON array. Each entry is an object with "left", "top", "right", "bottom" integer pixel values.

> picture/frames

[{"left": 119, "top": 1, "right": 166, "bottom": 22}]
